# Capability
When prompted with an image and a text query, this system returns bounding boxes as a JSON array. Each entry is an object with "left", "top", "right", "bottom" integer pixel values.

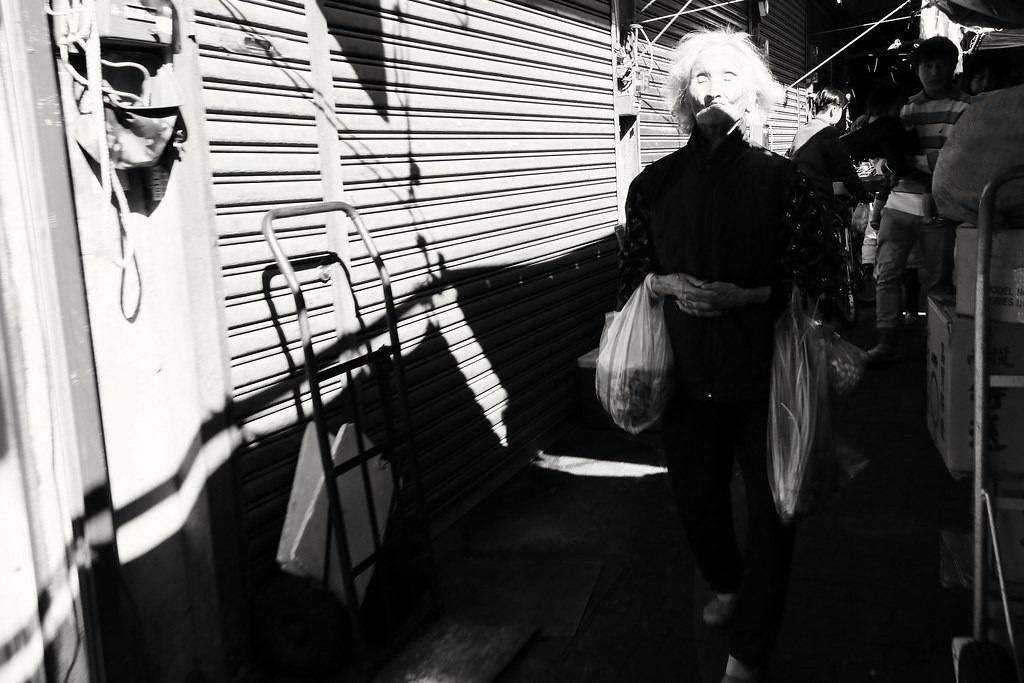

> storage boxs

[{"left": 925, "top": 220, "right": 1024, "bottom": 480}]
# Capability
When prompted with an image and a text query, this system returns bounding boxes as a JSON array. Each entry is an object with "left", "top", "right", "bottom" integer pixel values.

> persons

[
  {"left": 862, "top": 37, "right": 994, "bottom": 362},
  {"left": 790, "top": 87, "right": 875, "bottom": 211},
  {"left": 617, "top": 27, "right": 834, "bottom": 683}
]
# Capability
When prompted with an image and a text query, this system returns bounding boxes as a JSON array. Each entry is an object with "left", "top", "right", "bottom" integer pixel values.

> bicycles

[{"left": 820, "top": 193, "right": 877, "bottom": 328}]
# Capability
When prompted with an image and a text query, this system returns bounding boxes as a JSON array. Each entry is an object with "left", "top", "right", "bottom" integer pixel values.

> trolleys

[
  {"left": 939, "top": 172, "right": 1024, "bottom": 683},
  {"left": 261, "top": 202, "right": 464, "bottom": 683}
]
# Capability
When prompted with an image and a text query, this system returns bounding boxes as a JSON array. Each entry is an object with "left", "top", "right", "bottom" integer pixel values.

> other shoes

[
  {"left": 702, "top": 593, "right": 735, "bottom": 626},
  {"left": 897, "top": 309, "right": 919, "bottom": 327}
]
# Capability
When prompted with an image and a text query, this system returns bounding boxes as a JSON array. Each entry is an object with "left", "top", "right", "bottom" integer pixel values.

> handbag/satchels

[
  {"left": 765, "top": 317, "right": 872, "bottom": 521},
  {"left": 595, "top": 283, "right": 673, "bottom": 433}
]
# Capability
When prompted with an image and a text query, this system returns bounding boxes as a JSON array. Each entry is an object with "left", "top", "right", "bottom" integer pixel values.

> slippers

[{"left": 863, "top": 348, "right": 901, "bottom": 361}]
